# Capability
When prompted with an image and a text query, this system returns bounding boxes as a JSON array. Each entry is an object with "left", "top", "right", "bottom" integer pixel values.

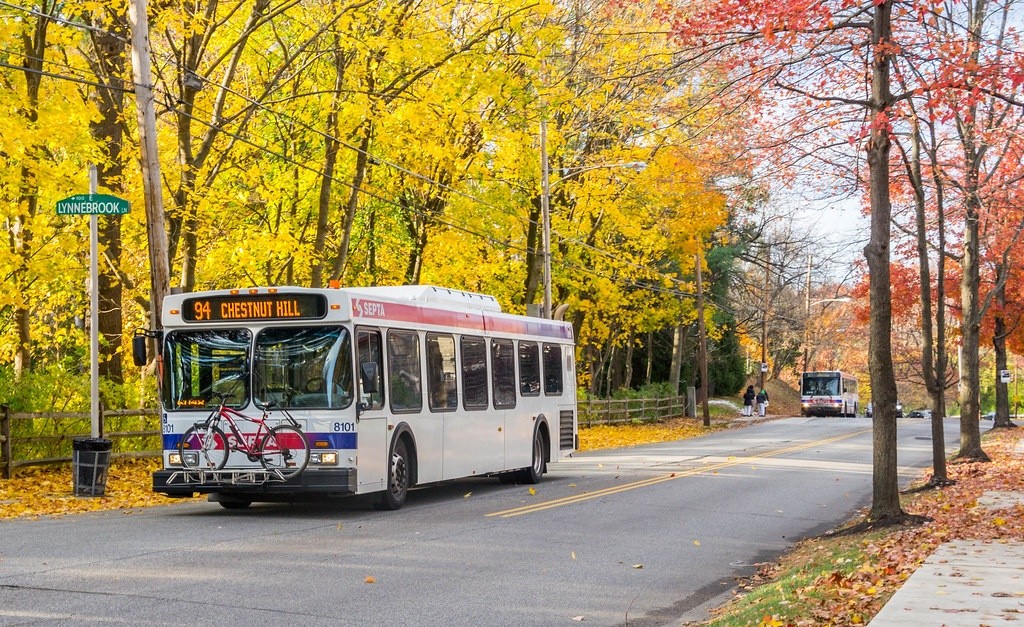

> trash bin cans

[{"left": 72, "top": 437, "right": 113, "bottom": 497}]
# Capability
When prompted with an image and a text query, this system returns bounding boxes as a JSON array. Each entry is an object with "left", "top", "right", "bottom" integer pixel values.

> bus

[
  {"left": 800, "top": 371, "right": 858, "bottom": 419},
  {"left": 131, "top": 286, "right": 578, "bottom": 512}
]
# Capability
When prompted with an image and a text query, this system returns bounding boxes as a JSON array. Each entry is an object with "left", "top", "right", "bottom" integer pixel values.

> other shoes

[{"left": 743, "top": 415, "right": 752, "bottom": 417}]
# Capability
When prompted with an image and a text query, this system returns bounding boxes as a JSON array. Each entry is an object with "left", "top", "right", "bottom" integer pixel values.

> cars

[
  {"left": 979, "top": 411, "right": 997, "bottom": 421},
  {"left": 906, "top": 410, "right": 932, "bottom": 419},
  {"left": 864, "top": 402, "right": 873, "bottom": 418},
  {"left": 895, "top": 400, "right": 903, "bottom": 418}
]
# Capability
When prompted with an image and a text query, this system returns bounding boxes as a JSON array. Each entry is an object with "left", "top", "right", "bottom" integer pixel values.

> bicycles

[{"left": 178, "top": 391, "right": 311, "bottom": 477}]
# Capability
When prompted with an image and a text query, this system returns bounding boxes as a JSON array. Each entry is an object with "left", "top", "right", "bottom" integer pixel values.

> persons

[
  {"left": 747, "top": 385, "right": 757, "bottom": 416},
  {"left": 756, "top": 390, "right": 766, "bottom": 417},
  {"left": 760, "top": 388, "right": 770, "bottom": 414},
  {"left": 743, "top": 389, "right": 754, "bottom": 417}
]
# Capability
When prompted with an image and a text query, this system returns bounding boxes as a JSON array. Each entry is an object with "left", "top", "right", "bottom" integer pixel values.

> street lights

[
  {"left": 803, "top": 298, "right": 853, "bottom": 371},
  {"left": 540, "top": 162, "right": 646, "bottom": 319}
]
